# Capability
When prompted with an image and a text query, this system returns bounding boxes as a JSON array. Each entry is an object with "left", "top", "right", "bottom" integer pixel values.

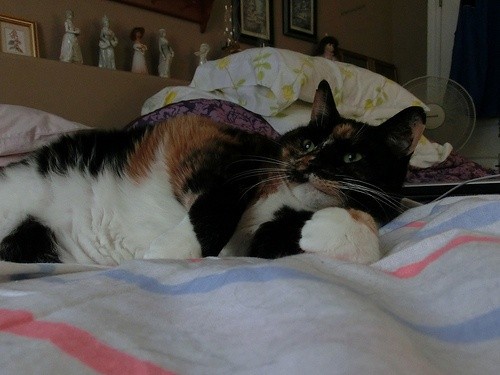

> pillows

[{"left": 0, "top": 47, "right": 454, "bottom": 168}]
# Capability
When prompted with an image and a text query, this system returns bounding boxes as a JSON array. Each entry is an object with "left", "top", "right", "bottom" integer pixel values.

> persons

[
  {"left": 311, "top": 36, "right": 339, "bottom": 61},
  {"left": 193, "top": 43, "right": 210, "bottom": 64},
  {"left": 130, "top": 27, "right": 149, "bottom": 75},
  {"left": 155, "top": 28, "right": 174, "bottom": 78},
  {"left": 59, "top": 10, "right": 84, "bottom": 64},
  {"left": 98, "top": 14, "right": 118, "bottom": 70}
]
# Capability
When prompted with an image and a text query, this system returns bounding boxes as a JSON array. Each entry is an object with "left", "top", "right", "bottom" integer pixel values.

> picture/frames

[
  {"left": 282, "top": 0, "right": 318, "bottom": 43},
  {"left": 233, "top": 0, "right": 274, "bottom": 46},
  {"left": 0, "top": 15, "right": 41, "bottom": 58},
  {"left": 338, "top": 48, "right": 400, "bottom": 84}
]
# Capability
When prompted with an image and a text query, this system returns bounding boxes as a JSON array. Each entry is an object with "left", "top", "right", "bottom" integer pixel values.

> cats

[{"left": 0, "top": 77, "right": 430, "bottom": 267}]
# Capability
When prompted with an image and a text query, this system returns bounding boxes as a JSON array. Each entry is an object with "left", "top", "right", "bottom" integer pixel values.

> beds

[{"left": 0, "top": 53, "right": 499, "bottom": 374}]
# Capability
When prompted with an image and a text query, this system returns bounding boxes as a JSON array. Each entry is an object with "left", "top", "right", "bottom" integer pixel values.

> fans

[{"left": 402, "top": 75, "right": 476, "bottom": 157}]
